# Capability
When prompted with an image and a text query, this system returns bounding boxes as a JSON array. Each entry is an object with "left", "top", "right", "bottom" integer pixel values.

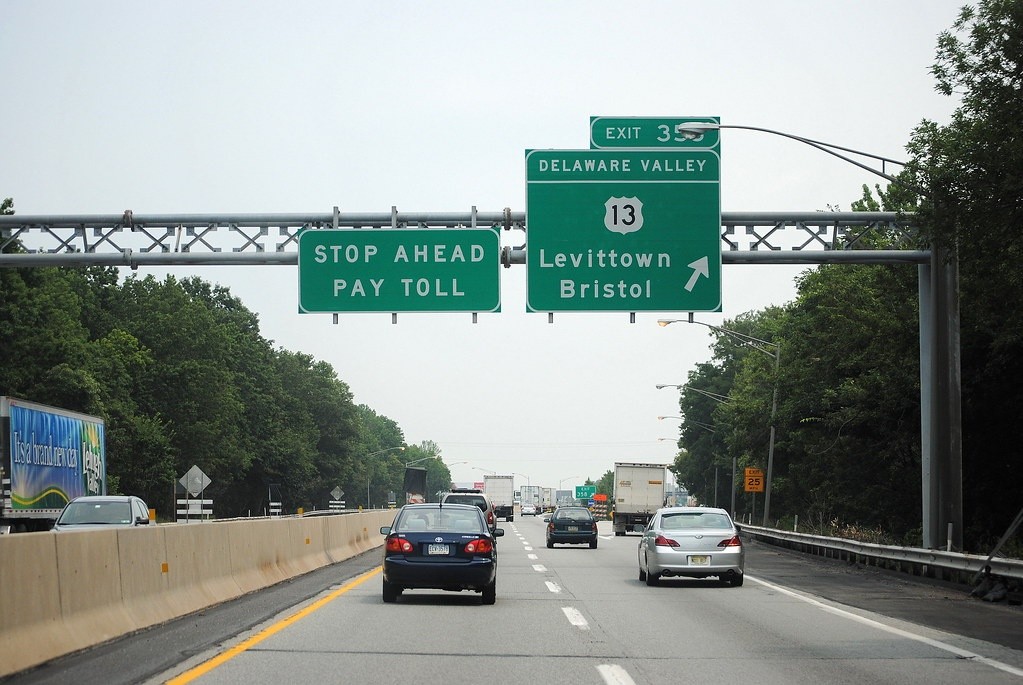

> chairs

[
  {"left": 456, "top": 520, "right": 472, "bottom": 531},
  {"left": 408, "top": 519, "right": 427, "bottom": 531}
]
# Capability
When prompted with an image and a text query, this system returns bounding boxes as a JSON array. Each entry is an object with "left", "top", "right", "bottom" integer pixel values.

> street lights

[
  {"left": 675, "top": 120, "right": 944, "bottom": 553},
  {"left": 657, "top": 317, "right": 781, "bottom": 526},
  {"left": 512, "top": 472, "right": 530, "bottom": 485},
  {"left": 654, "top": 380, "right": 759, "bottom": 524},
  {"left": 560, "top": 476, "right": 580, "bottom": 500}
]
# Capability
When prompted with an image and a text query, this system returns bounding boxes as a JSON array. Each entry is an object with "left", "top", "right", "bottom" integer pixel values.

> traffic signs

[
  {"left": 576, "top": 486, "right": 596, "bottom": 499},
  {"left": 524, "top": 146, "right": 723, "bottom": 313}
]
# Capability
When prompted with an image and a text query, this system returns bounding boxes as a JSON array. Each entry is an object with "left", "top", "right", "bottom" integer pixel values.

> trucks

[
  {"left": 483, "top": 475, "right": 515, "bottom": 523},
  {"left": 519, "top": 484, "right": 556, "bottom": 515},
  {"left": 610, "top": 462, "right": 668, "bottom": 538}
]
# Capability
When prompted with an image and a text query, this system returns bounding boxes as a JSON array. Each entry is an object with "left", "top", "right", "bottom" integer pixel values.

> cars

[
  {"left": 50, "top": 495, "right": 156, "bottom": 531},
  {"left": 637, "top": 508, "right": 746, "bottom": 587},
  {"left": 544, "top": 507, "right": 599, "bottom": 550},
  {"left": 380, "top": 504, "right": 505, "bottom": 605},
  {"left": 521, "top": 503, "right": 537, "bottom": 517}
]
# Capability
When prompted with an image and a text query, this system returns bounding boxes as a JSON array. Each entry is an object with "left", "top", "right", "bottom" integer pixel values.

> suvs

[{"left": 441, "top": 490, "right": 498, "bottom": 542}]
